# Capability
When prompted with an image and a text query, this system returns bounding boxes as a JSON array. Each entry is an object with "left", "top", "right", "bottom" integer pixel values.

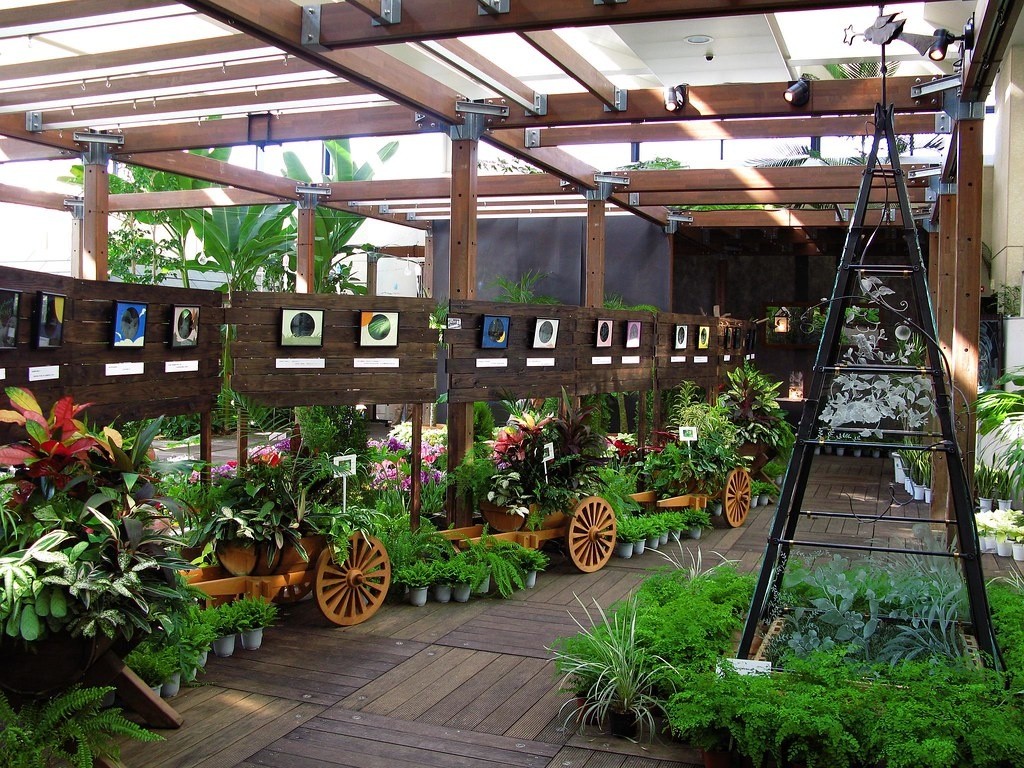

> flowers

[{"left": 133, "top": 398, "right": 552, "bottom": 523}]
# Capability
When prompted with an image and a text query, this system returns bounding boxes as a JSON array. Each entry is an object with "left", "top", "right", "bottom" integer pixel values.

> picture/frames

[{"left": 0, "top": 289, "right": 746, "bottom": 353}]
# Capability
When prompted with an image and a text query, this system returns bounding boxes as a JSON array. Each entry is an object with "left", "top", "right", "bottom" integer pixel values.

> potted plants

[
  {"left": 121, "top": 594, "right": 280, "bottom": 697},
  {"left": 540, "top": 589, "right": 667, "bottom": 746},
  {"left": 811, "top": 430, "right": 889, "bottom": 458},
  {"left": 615, "top": 506, "right": 713, "bottom": 560},
  {"left": 891, "top": 432, "right": 1024, "bottom": 562},
  {"left": 715, "top": 460, "right": 787, "bottom": 516},
  {"left": 353, "top": 522, "right": 549, "bottom": 607}
]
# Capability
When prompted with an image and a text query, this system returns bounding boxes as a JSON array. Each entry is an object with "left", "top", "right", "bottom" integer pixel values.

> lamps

[
  {"left": 663, "top": 85, "right": 685, "bottom": 112},
  {"left": 773, "top": 306, "right": 791, "bottom": 333},
  {"left": 783, "top": 81, "right": 811, "bottom": 107},
  {"left": 928, "top": 11, "right": 975, "bottom": 61}
]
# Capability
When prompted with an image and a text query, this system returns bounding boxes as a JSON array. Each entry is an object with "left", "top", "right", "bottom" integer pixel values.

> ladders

[{"left": 732, "top": 100, "right": 1007, "bottom": 673}]
